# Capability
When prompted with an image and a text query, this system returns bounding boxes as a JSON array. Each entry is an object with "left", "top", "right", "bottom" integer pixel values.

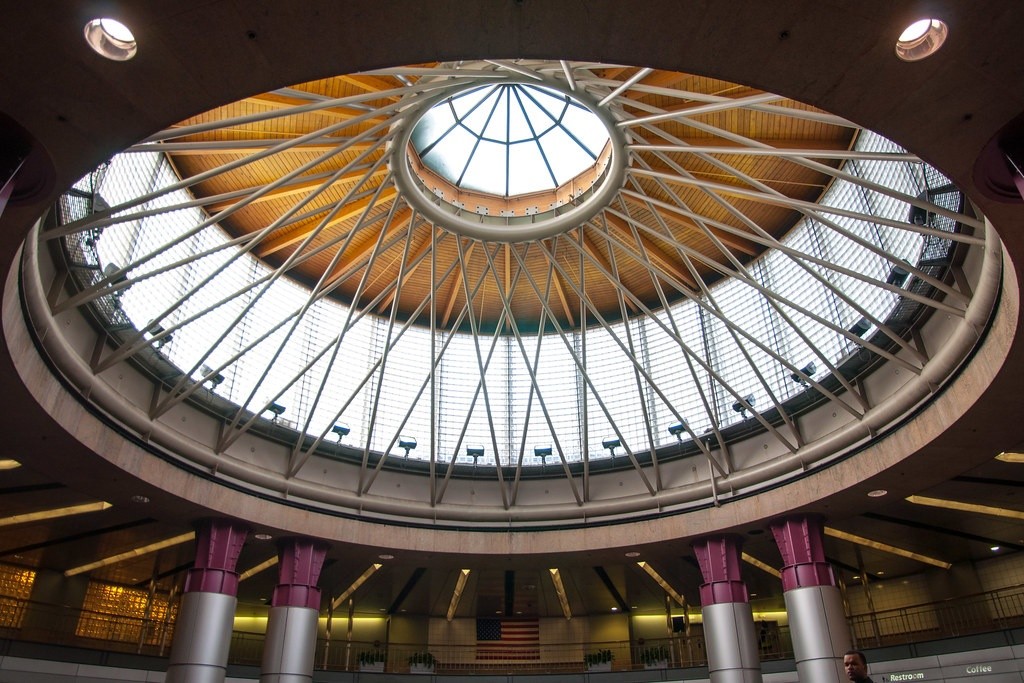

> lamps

[
  {"left": 791, "top": 361, "right": 817, "bottom": 388},
  {"left": 732, "top": 393, "right": 756, "bottom": 420},
  {"left": 668, "top": 418, "right": 691, "bottom": 443},
  {"left": 398, "top": 435, "right": 417, "bottom": 458},
  {"left": 200, "top": 363, "right": 226, "bottom": 390},
  {"left": 886, "top": 258, "right": 911, "bottom": 287},
  {"left": 909, "top": 184, "right": 957, "bottom": 226},
  {"left": 848, "top": 315, "right": 871, "bottom": 346},
  {"left": 147, "top": 318, "right": 174, "bottom": 349},
  {"left": 602, "top": 436, "right": 623, "bottom": 458},
  {"left": 104, "top": 261, "right": 132, "bottom": 298},
  {"left": 467, "top": 444, "right": 484, "bottom": 465},
  {"left": 66, "top": 187, "right": 114, "bottom": 241},
  {"left": 330, "top": 420, "right": 351, "bottom": 444},
  {"left": 265, "top": 400, "right": 286, "bottom": 422},
  {"left": 534, "top": 443, "right": 553, "bottom": 466}
]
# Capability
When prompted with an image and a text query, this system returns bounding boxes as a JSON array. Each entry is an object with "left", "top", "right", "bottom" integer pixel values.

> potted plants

[
  {"left": 584, "top": 648, "right": 615, "bottom": 671},
  {"left": 640, "top": 644, "right": 670, "bottom": 669},
  {"left": 357, "top": 651, "right": 384, "bottom": 673},
  {"left": 408, "top": 652, "right": 438, "bottom": 674}
]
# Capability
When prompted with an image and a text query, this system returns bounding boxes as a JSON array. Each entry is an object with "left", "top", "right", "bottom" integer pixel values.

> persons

[{"left": 844, "top": 651, "right": 874, "bottom": 683}]
